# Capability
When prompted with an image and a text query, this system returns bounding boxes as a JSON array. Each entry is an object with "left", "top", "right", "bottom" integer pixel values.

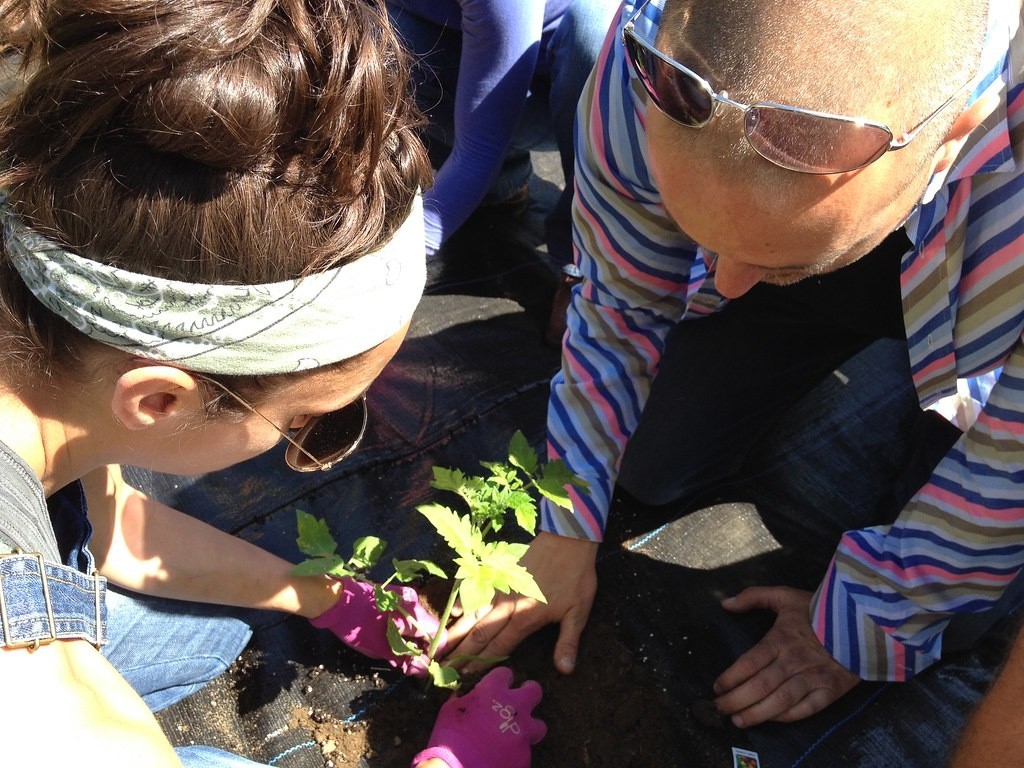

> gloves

[
  {"left": 308, "top": 576, "right": 439, "bottom": 678},
  {"left": 408, "top": 666, "right": 547, "bottom": 768}
]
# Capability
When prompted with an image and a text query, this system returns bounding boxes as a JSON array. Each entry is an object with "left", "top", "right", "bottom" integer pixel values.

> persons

[
  {"left": 428, "top": 0, "right": 1024, "bottom": 727},
  {"left": 0, "top": 0, "right": 622, "bottom": 768}
]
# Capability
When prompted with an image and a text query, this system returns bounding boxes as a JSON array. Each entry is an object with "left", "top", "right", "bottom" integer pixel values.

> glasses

[
  {"left": 619, "top": 1, "right": 942, "bottom": 175},
  {"left": 128, "top": 347, "right": 370, "bottom": 476}
]
